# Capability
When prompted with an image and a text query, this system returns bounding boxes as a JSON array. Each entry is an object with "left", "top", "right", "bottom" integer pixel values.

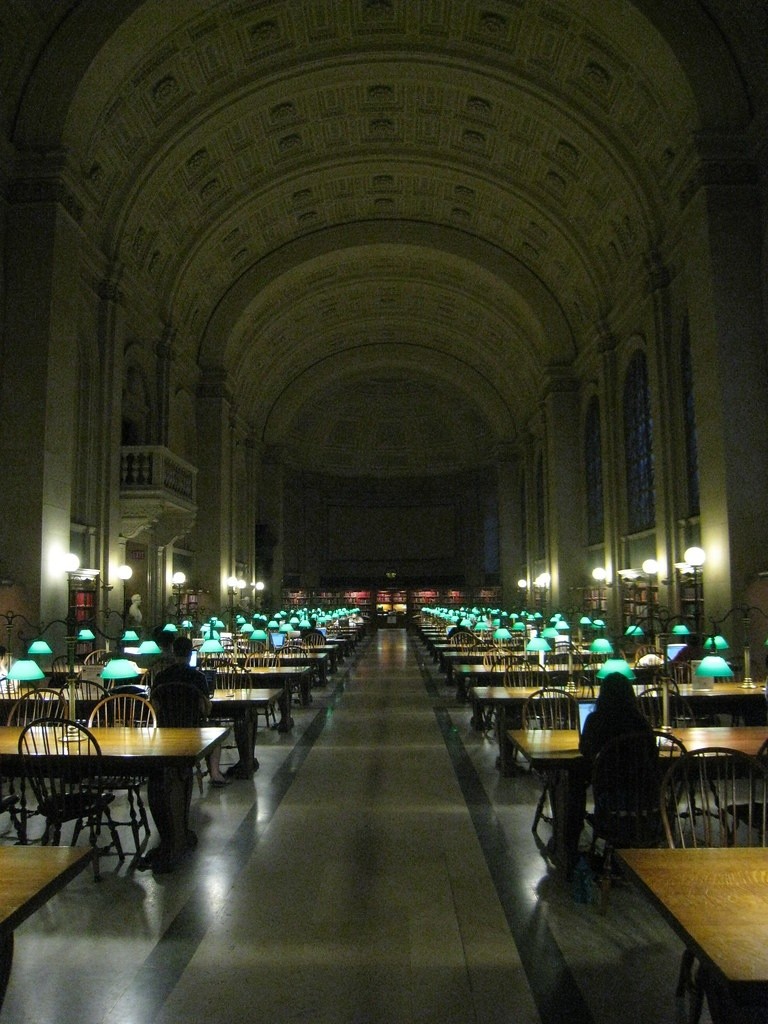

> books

[
  {"left": 75, "top": 592, "right": 95, "bottom": 621},
  {"left": 180, "top": 595, "right": 198, "bottom": 614},
  {"left": 289, "top": 592, "right": 304, "bottom": 604},
  {"left": 344, "top": 591, "right": 371, "bottom": 603},
  {"left": 623, "top": 580, "right": 658, "bottom": 631},
  {"left": 583, "top": 590, "right": 607, "bottom": 618},
  {"left": 445, "top": 591, "right": 465, "bottom": 604},
  {"left": 481, "top": 590, "right": 495, "bottom": 602},
  {"left": 680, "top": 575, "right": 697, "bottom": 631},
  {"left": 376, "top": 593, "right": 408, "bottom": 603},
  {"left": 412, "top": 591, "right": 438, "bottom": 603},
  {"left": 318, "top": 592, "right": 338, "bottom": 604}
]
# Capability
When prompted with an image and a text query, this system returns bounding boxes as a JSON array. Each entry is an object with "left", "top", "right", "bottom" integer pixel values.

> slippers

[{"left": 212, "top": 776, "right": 232, "bottom": 787}]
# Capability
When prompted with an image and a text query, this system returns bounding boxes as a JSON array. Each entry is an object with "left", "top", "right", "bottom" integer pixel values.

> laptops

[
  {"left": 446, "top": 625, "right": 457, "bottom": 635},
  {"left": 666, "top": 644, "right": 688, "bottom": 662},
  {"left": 189, "top": 648, "right": 199, "bottom": 670},
  {"left": 576, "top": 698, "right": 597, "bottom": 740},
  {"left": 201, "top": 669, "right": 216, "bottom": 698},
  {"left": 316, "top": 628, "right": 327, "bottom": 637},
  {"left": 338, "top": 620, "right": 350, "bottom": 627},
  {"left": 270, "top": 632, "right": 285, "bottom": 648}
]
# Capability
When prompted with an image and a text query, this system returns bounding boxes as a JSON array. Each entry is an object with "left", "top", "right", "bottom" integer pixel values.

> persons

[
  {"left": 128, "top": 594, "right": 142, "bottom": 623},
  {"left": 668, "top": 636, "right": 704, "bottom": 683},
  {"left": 447, "top": 618, "right": 483, "bottom": 644},
  {"left": 166, "top": 596, "right": 177, "bottom": 615},
  {"left": 301, "top": 619, "right": 326, "bottom": 644},
  {"left": 150, "top": 636, "right": 232, "bottom": 786},
  {"left": 578, "top": 672, "right": 660, "bottom": 876}
]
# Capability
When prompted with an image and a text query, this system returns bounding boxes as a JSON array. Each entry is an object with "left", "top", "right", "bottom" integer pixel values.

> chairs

[{"left": 0, "top": 614, "right": 768, "bottom": 1024}]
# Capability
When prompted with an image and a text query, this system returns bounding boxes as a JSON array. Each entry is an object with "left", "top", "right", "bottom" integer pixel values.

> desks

[
  {"left": 469, "top": 681, "right": 768, "bottom": 731},
  {"left": 442, "top": 649, "right": 617, "bottom": 687},
  {"left": 0, "top": 727, "right": 236, "bottom": 873},
  {"left": 432, "top": 643, "right": 526, "bottom": 667},
  {"left": 325, "top": 633, "right": 356, "bottom": 652},
  {"left": 41, "top": 664, "right": 311, "bottom": 733},
  {"left": 506, "top": 728, "right": 768, "bottom": 866},
  {"left": 0, "top": 843, "right": 93, "bottom": 1014},
  {"left": 0, "top": 686, "right": 283, "bottom": 781},
  {"left": 287, "top": 638, "right": 349, "bottom": 657},
  {"left": 421, "top": 629, "right": 447, "bottom": 642},
  {"left": 451, "top": 660, "right": 734, "bottom": 704},
  {"left": 424, "top": 632, "right": 448, "bottom": 646},
  {"left": 220, "top": 644, "right": 339, "bottom": 675},
  {"left": 196, "top": 652, "right": 328, "bottom": 688},
  {"left": 616, "top": 847, "right": 768, "bottom": 1024},
  {"left": 428, "top": 636, "right": 541, "bottom": 659}
]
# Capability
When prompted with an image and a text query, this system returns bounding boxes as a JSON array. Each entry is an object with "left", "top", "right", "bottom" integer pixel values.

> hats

[{"left": 173, "top": 637, "right": 197, "bottom": 651}]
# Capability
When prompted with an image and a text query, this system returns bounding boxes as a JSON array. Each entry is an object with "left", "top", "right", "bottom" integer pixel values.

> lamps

[{"left": 0, "top": 545, "right": 768, "bottom": 700}]
